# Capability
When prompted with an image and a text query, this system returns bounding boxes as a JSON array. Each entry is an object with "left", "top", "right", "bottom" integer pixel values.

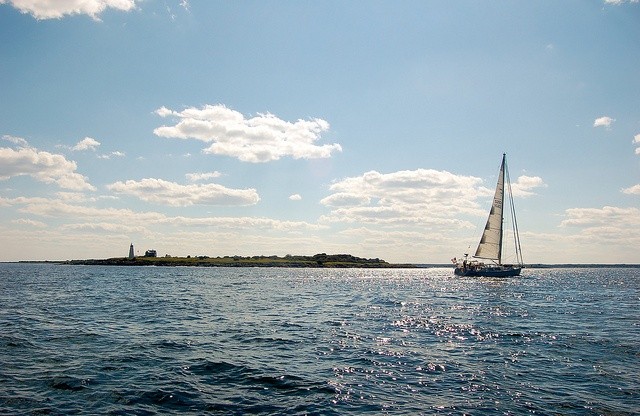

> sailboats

[{"left": 450, "top": 151, "right": 526, "bottom": 279}]
